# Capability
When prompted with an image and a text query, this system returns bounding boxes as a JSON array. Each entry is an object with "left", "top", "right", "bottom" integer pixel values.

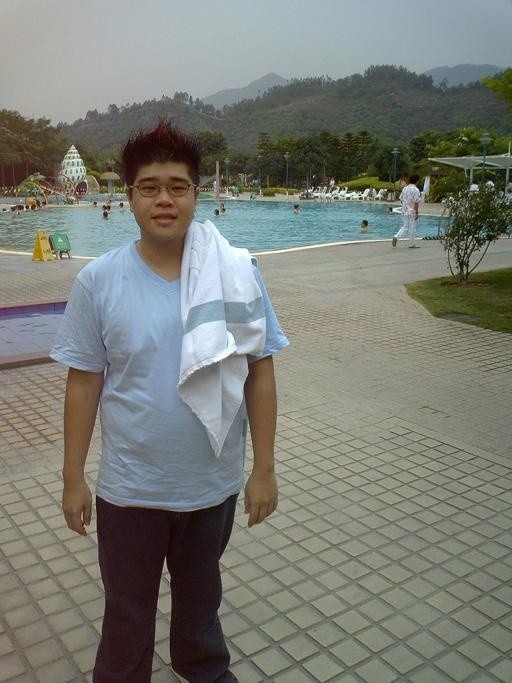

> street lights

[
  {"left": 283, "top": 151, "right": 290, "bottom": 187},
  {"left": 478, "top": 132, "right": 491, "bottom": 186},
  {"left": 391, "top": 148, "right": 401, "bottom": 192},
  {"left": 224, "top": 158, "right": 230, "bottom": 189},
  {"left": 256, "top": 155, "right": 264, "bottom": 187}
]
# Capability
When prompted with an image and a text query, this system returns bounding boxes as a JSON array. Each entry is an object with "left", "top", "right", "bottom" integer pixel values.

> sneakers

[{"left": 393, "top": 238, "right": 397, "bottom": 246}]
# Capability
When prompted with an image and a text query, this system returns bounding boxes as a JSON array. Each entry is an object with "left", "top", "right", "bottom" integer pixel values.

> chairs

[{"left": 313, "top": 186, "right": 388, "bottom": 201}]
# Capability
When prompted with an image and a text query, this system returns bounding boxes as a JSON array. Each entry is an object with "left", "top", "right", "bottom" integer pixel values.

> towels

[{"left": 176, "top": 220, "right": 270, "bottom": 461}]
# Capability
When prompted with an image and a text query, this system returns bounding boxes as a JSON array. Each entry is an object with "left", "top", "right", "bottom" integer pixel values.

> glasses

[{"left": 130, "top": 183, "right": 197, "bottom": 197}]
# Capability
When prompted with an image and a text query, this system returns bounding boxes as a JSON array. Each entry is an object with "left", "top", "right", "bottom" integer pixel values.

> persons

[
  {"left": 386, "top": 207, "right": 392, "bottom": 215},
  {"left": 92, "top": 200, "right": 124, "bottom": 218},
  {"left": 293, "top": 204, "right": 302, "bottom": 214},
  {"left": 212, "top": 178, "right": 221, "bottom": 196},
  {"left": 214, "top": 204, "right": 226, "bottom": 216},
  {"left": 49, "top": 113, "right": 291, "bottom": 683},
  {"left": 469, "top": 178, "right": 494, "bottom": 197},
  {"left": 3, "top": 201, "right": 46, "bottom": 215},
  {"left": 312, "top": 177, "right": 336, "bottom": 193},
  {"left": 392, "top": 175, "right": 421, "bottom": 248},
  {"left": 360, "top": 220, "right": 368, "bottom": 232}
]
